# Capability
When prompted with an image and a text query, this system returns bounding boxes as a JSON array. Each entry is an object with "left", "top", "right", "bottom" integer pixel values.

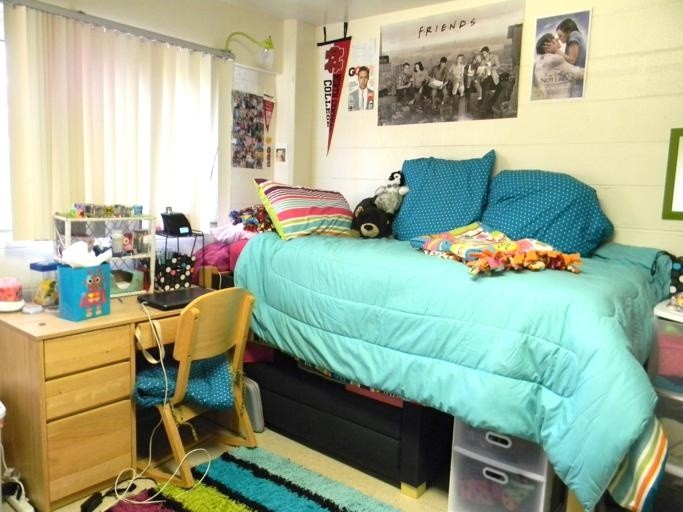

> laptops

[{"left": 137, "top": 287, "right": 213, "bottom": 311}]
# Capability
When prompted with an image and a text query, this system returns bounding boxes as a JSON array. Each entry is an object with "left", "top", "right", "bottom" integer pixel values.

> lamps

[{"left": 222, "top": 28, "right": 274, "bottom": 70}]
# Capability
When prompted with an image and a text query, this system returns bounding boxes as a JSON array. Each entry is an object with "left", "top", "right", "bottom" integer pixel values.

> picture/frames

[{"left": 661, "top": 128, "right": 683, "bottom": 219}]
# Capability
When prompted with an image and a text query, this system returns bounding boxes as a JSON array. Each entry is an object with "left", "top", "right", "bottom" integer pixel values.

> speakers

[{"left": 161, "top": 212, "right": 192, "bottom": 236}]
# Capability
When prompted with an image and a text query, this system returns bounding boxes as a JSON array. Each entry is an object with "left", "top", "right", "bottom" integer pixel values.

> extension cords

[{"left": 1, "top": 479, "right": 34, "bottom": 512}]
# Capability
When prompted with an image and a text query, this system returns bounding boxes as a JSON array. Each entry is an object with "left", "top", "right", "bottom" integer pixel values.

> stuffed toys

[
  {"left": 351, "top": 193, "right": 393, "bottom": 238},
  {"left": 372, "top": 171, "right": 409, "bottom": 222}
]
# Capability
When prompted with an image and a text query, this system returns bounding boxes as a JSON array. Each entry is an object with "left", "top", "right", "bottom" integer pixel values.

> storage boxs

[
  {"left": 654, "top": 294, "right": 681, "bottom": 377},
  {"left": 445, "top": 413, "right": 554, "bottom": 512}
]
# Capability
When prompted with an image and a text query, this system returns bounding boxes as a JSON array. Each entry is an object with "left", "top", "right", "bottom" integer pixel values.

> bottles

[{"left": 111, "top": 229, "right": 123, "bottom": 256}]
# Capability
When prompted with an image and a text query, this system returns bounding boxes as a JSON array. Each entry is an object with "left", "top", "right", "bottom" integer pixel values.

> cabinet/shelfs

[
  {"left": 53, "top": 216, "right": 158, "bottom": 299},
  {"left": 651, "top": 386, "right": 683, "bottom": 480},
  {"left": 246, "top": 364, "right": 427, "bottom": 488}
]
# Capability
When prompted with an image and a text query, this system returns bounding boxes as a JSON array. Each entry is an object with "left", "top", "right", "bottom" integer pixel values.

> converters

[{"left": 82, "top": 492, "right": 102, "bottom": 512}]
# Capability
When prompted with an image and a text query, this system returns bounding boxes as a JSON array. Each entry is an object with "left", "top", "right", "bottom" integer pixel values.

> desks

[{"left": 1, "top": 296, "right": 183, "bottom": 512}]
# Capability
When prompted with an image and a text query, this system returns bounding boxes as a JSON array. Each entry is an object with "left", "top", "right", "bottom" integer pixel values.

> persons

[
  {"left": 535, "top": 33, "right": 584, "bottom": 98},
  {"left": 395, "top": 48, "right": 501, "bottom": 114},
  {"left": 348, "top": 66, "right": 374, "bottom": 111},
  {"left": 276, "top": 149, "right": 285, "bottom": 162},
  {"left": 542, "top": 18, "right": 587, "bottom": 97}
]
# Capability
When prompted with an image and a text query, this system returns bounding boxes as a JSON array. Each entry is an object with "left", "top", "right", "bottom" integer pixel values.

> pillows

[{"left": 252, "top": 177, "right": 360, "bottom": 240}]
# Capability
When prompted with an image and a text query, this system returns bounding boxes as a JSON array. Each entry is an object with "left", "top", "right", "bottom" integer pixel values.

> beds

[{"left": 213, "top": 222, "right": 674, "bottom": 511}]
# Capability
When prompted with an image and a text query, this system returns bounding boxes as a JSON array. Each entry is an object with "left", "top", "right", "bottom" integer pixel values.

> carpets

[{"left": 103, "top": 447, "right": 404, "bottom": 511}]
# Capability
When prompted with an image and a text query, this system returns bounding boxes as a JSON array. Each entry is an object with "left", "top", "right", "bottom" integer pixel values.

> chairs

[{"left": 137, "top": 289, "right": 255, "bottom": 488}]
